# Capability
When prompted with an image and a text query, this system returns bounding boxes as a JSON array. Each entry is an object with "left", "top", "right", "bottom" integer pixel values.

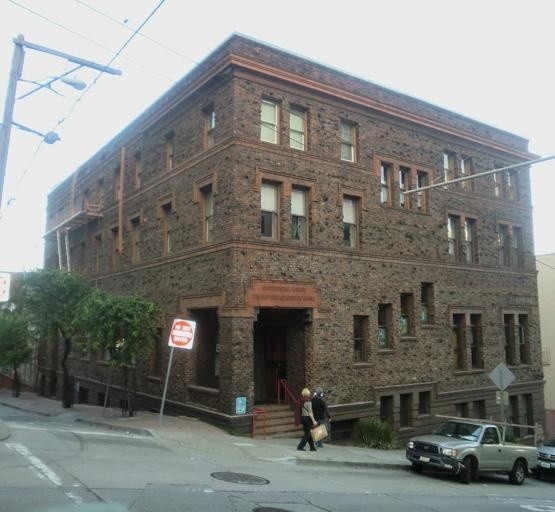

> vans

[{"left": 530, "top": 435, "right": 555, "bottom": 481}]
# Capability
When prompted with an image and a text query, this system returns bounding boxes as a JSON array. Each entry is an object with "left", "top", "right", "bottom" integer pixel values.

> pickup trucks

[{"left": 405, "top": 416, "right": 539, "bottom": 486}]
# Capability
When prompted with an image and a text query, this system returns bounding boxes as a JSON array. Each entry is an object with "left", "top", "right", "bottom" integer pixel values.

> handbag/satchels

[{"left": 311, "top": 423, "right": 328, "bottom": 441}]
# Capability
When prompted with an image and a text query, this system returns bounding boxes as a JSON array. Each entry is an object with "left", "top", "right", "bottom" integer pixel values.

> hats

[
  {"left": 317, "top": 388, "right": 323, "bottom": 396},
  {"left": 302, "top": 388, "right": 310, "bottom": 397}
]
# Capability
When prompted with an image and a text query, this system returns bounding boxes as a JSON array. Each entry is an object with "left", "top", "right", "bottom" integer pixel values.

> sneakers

[{"left": 297, "top": 442, "right": 323, "bottom": 450}]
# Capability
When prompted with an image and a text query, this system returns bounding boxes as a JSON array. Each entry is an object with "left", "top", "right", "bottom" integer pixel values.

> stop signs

[{"left": 167, "top": 318, "right": 198, "bottom": 350}]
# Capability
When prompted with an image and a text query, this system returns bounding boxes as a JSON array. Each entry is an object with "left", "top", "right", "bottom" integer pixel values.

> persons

[
  {"left": 296, "top": 388, "right": 318, "bottom": 452},
  {"left": 311, "top": 387, "right": 332, "bottom": 448}
]
